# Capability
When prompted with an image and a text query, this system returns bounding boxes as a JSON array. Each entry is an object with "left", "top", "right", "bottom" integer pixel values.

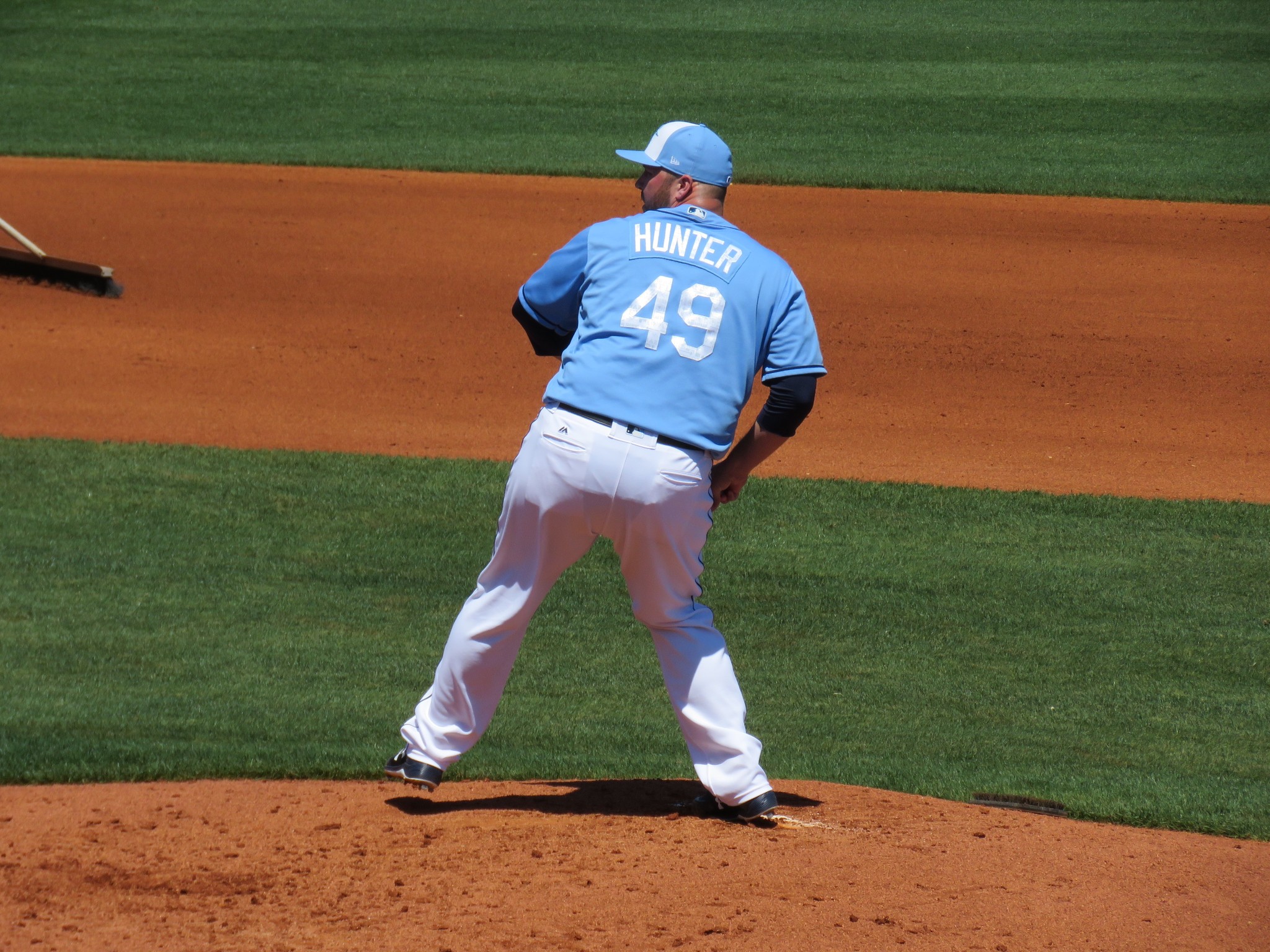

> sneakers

[
  {"left": 383, "top": 748, "right": 443, "bottom": 793},
  {"left": 709, "top": 786, "right": 779, "bottom": 820}
]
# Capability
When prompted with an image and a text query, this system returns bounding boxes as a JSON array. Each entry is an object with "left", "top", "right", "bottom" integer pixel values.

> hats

[{"left": 615, "top": 121, "right": 733, "bottom": 187}]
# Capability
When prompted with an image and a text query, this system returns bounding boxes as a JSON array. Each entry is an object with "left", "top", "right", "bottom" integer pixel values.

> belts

[{"left": 558, "top": 402, "right": 703, "bottom": 452}]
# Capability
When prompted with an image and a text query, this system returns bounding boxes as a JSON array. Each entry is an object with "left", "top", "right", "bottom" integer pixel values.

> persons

[{"left": 382, "top": 120, "right": 826, "bottom": 822}]
